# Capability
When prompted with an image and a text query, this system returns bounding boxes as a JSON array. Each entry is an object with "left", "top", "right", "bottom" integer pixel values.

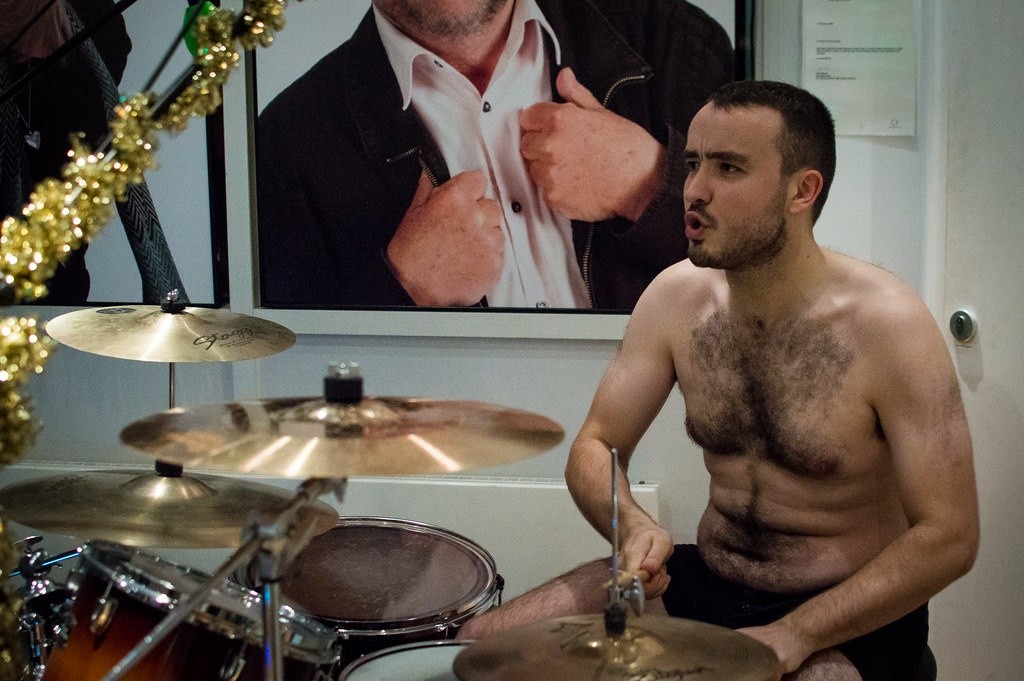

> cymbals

[
  {"left": 44, "top": 303, "right": 297, "bottom": 363},
  {"left": 452, "top": 612, "right": 786, "bottom": 681},
  {"left": 0, "top": 392, "right": 566, "bottom": 551}
]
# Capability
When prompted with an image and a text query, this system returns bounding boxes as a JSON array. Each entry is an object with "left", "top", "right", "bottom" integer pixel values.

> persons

[
  {"left": 450, "top": 78, "right": 979, "bottom": 681},
  {"left": 255, "top": 1, "right": 736, "bottom": 317}
]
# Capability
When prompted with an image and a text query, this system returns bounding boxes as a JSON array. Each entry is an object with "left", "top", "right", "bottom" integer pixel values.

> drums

[{"left": 36, "top": 517, "right": 502, "bottom": 680}]
